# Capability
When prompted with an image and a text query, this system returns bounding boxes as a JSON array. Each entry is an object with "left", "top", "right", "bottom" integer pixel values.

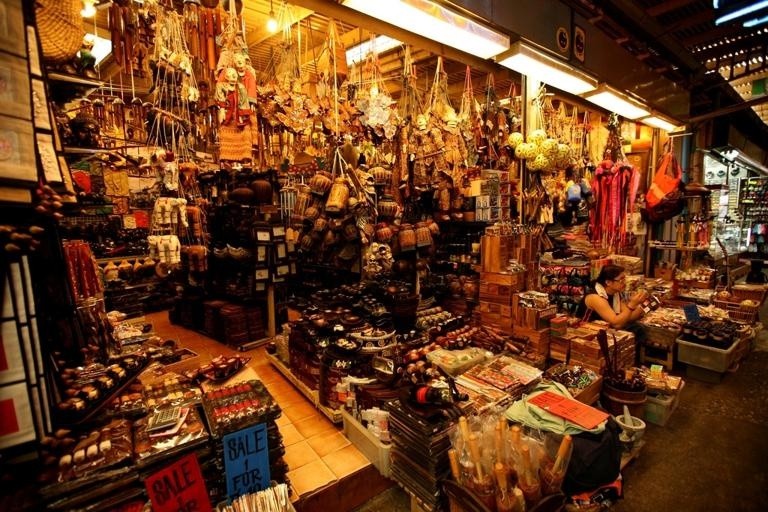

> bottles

[
  {"left": 409, "top": 383, "right": 449, "bottom": 407},
  {"left": 446, "top": 385, "right": 469, "bottom": 405}
]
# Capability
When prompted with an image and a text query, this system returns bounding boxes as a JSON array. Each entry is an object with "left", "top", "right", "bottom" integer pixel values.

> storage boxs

[
  {"left": 341, "top": 399, "right": 395, "bottom": 479},
  {"left": 646, "top": 380, "right": 685, "bottom": 426},
  {"left": 676, "top": 331, "right": 739, "bottom": 372}
]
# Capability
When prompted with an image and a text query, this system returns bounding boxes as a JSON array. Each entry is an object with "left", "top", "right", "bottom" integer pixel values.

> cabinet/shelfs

[
  {"left": 737, "top": 177, "right": 767, "bottom": 236},
  {"left": 703, "top": 183, "right": 729, "bottom": 225}
]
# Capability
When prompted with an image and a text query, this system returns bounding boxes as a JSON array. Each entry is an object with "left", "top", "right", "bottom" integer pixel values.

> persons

[{"left": 576, "top": 264, "right": 647, "bottom": 330}]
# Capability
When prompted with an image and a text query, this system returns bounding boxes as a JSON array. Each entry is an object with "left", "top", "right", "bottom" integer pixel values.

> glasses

[{"left": 613, "top": 278, "right": 626, "bottom": 284}]
